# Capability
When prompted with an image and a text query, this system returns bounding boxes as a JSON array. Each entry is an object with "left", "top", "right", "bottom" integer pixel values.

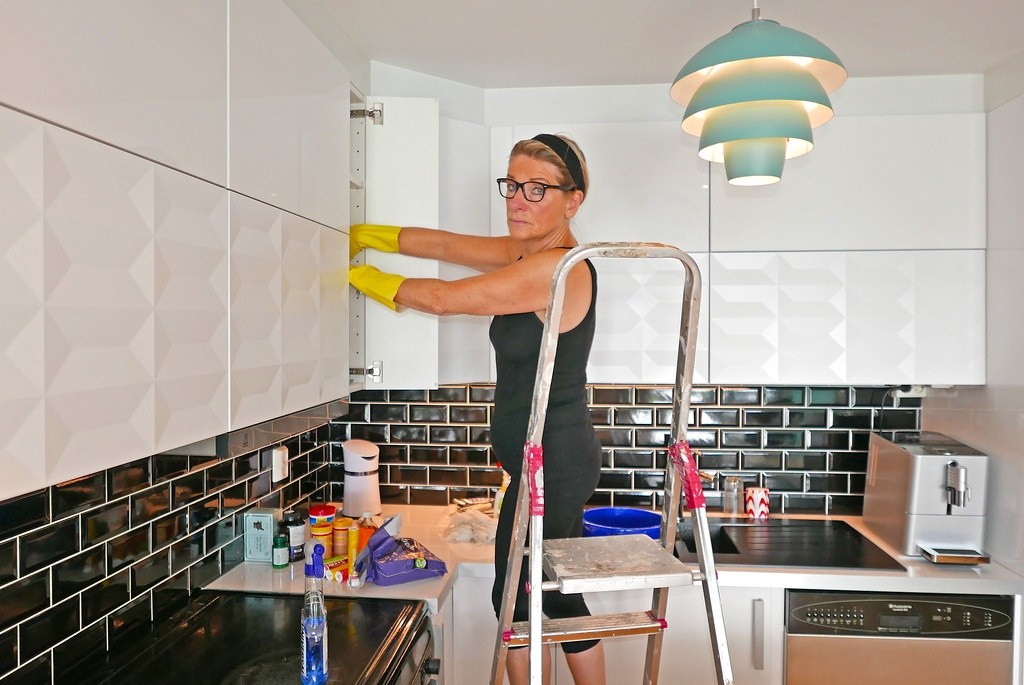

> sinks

[{"left": 675, "top": 517, "right": 744, "bottom": 560}]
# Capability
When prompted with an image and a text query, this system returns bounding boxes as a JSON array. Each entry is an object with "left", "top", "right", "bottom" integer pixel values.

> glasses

[{"left": 497, "top": 178, "right": 583, "bottom": 202}]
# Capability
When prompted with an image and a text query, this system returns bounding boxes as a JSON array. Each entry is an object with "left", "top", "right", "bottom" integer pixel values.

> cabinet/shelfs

[
  {"left": 489, "top": 72, "right": 987, "bottom": 387},
  {"left": 0, "top": 0, "right": 439, "bottom": 503},
  {"left": 432, "top": 577, "right": 784, "bottom": 685}
]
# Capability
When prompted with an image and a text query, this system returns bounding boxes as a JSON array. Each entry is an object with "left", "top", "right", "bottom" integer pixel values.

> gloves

[
  {"left": 349, "top": 223, "right": 403, "bottom": 260},
  {"left": 349, "top": 264, "right": 410, "bottom": 313}
]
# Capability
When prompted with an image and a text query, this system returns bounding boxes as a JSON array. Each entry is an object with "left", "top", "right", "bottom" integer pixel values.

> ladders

[{"left": 489, "top": 239, "right": 734, "bottom": 684}]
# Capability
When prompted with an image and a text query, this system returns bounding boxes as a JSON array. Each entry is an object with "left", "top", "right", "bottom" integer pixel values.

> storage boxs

[{"left": 244, "top": 507, "right": 283, "bottom": 565}]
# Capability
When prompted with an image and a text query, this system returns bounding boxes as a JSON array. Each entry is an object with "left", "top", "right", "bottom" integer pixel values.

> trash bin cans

[{"left": 582, "top": 506, "right": 663, "bottom": 539}]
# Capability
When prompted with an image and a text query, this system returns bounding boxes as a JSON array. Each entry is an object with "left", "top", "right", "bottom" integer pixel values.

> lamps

[{"left": 669, "top": 0, "right": 849, "bottom": 185}]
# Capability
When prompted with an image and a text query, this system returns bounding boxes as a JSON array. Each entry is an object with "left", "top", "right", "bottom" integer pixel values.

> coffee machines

[{"left": 863, "top": 429, "right": 991, "bottom": 564}]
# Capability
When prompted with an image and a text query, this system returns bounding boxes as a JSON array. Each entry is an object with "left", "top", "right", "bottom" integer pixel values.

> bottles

[
  {"left": 301, "top": 538, "right": 328, "bottom": 685},
  {"left": 358, "top": 512, "right": 378, "bottom": 556},
  {"left": 272, "top": 510, "right": 305, "bottom": 569}
]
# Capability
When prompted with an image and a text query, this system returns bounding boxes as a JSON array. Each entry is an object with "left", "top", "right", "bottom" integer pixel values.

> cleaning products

[{"left": 297, "top": 538, "right": 331, "bottom": 684}]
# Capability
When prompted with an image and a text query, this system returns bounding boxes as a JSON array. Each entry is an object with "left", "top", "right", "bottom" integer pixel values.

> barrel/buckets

[{"left": 582, "top": 508, "right": 663, "bottom": 540}]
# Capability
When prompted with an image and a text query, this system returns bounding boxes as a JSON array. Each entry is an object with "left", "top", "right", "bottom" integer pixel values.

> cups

[
  {"left": 723, "top": 477, "right": 744, "bottom": 514},
  {"left": 746, "top": 487, "right": 769, "bottom": 518}
]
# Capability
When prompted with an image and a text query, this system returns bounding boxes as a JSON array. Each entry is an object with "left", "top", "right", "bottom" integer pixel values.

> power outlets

[{"left": 272, "top": 446, "right": 289, "bottom": 483}]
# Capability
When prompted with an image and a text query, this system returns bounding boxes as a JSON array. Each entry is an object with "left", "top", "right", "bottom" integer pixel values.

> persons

[{"left": 349, "top": 133, "right": 607, "bottom": 685}]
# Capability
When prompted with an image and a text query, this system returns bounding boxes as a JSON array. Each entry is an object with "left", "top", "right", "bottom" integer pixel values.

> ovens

[{"left": 784, "top": 588, "right": 1015, "bottom": 685}]
